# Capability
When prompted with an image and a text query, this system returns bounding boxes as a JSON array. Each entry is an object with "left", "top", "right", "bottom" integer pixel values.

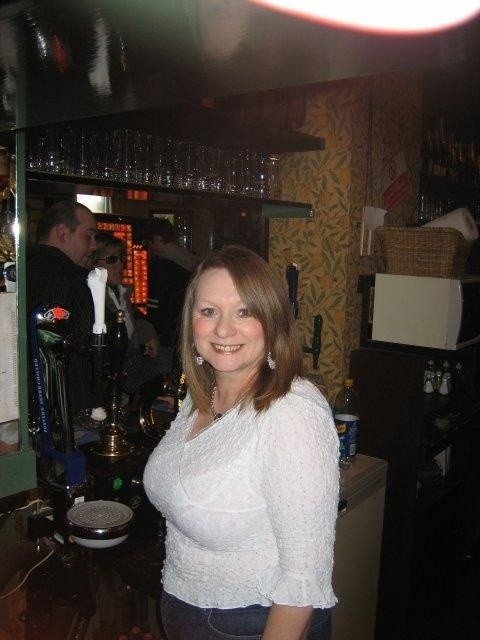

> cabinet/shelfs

[{"left": 26, "top": 106, "right": 325, "bottom": 219}]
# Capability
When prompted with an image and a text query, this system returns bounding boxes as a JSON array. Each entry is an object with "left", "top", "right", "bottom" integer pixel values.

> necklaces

[{"left": 208, "top": 382, "right": 243, "bottom": 420}]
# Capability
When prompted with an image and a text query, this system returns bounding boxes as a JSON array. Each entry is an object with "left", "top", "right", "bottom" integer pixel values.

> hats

[{"left": 135, "top": 217, "right": 171, "bottom": 239}]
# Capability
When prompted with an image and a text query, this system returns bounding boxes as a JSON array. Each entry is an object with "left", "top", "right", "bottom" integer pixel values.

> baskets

[{"left": 375, "top": 227, "right": 476, "bottom": 279}]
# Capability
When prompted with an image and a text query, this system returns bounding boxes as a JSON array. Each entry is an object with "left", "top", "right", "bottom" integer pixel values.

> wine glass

[{"left": 24, "top": 123, "right": 285, "bottom": 200}]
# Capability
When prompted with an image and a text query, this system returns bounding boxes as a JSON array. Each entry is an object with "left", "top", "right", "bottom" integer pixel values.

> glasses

[{"left": 98, "top": 255, "right": 127, "bottom": 264}]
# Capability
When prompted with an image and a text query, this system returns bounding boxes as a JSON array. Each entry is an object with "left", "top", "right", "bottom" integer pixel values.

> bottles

[
  {"left": 421, "top": 110, "right": 480, "bottom": 186},
  {"left": 330, "top": 378, "right": 363, "bottom": 470},
  {"left": 420, "top": 193, "right": 480, "bottom": 225},
  {"left": 1, "top": 144, "right": 17, "bottom": 293},
  {"left": 421, "top": 358, "right": 466, "bottom": 428}
]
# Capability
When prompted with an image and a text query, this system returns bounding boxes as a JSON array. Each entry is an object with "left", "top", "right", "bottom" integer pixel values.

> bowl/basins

[{"left": 66, "top": 498, "right": 137, "bottom": 550}]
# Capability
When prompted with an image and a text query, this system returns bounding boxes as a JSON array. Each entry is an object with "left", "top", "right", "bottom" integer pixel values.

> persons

[
  {"left": 90, "top": 227, "right": 162, "bottom": 412},
  {"left": 143, "top": 243, "right": 342, "bottom": 640},
  {"left": 26, "top": 195, "right": 109, "bottom": 413},
  {"left": 140, "top": 218, "right": 199, "bottom": 382}
]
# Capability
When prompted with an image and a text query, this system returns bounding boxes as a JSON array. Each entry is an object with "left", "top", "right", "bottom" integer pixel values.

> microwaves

[{"left": 370, "top": 272, "right": 479, "bottom": 352}]
